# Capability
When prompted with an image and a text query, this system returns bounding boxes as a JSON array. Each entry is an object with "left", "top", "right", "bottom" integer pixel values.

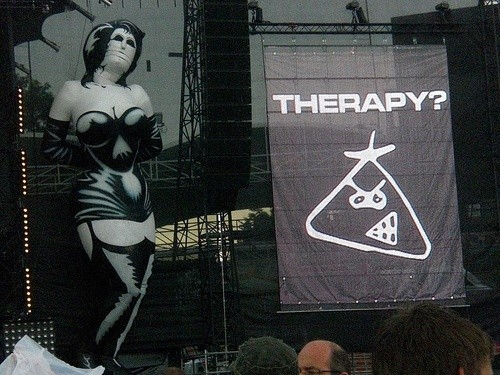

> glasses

[{"left": 298, "top": 367, "right": 341, "bottom": 375}]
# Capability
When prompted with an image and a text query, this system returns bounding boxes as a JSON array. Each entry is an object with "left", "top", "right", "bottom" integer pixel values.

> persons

[
  {"left": 372, "top": 301, "right": 494, "bottom": 375},
  {"left": 229, "top": 335, "right": 298, "bottom": 375},
  {"left": 298, "top": 339, "right": 351, "bottom": 375},
  {"left": 40, "top": 18, "right": 162, "bottom": 375}
]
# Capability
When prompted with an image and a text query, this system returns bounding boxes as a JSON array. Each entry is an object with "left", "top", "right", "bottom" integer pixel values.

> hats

[{"left": 234, "top": 336, "right": 298, "bottom": 375}]
